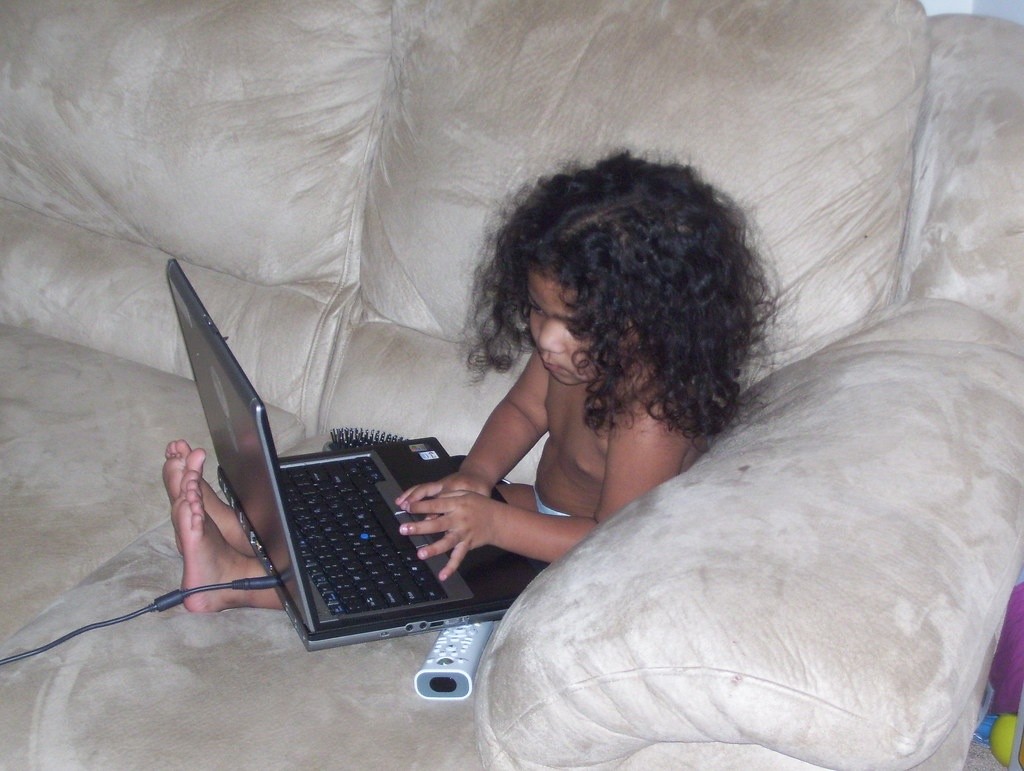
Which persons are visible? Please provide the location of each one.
[163,148,776,613]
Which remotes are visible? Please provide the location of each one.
[413,621,495,701]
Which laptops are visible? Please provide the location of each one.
[167,259,553,651]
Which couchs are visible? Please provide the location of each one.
[0,0,1024,771]
